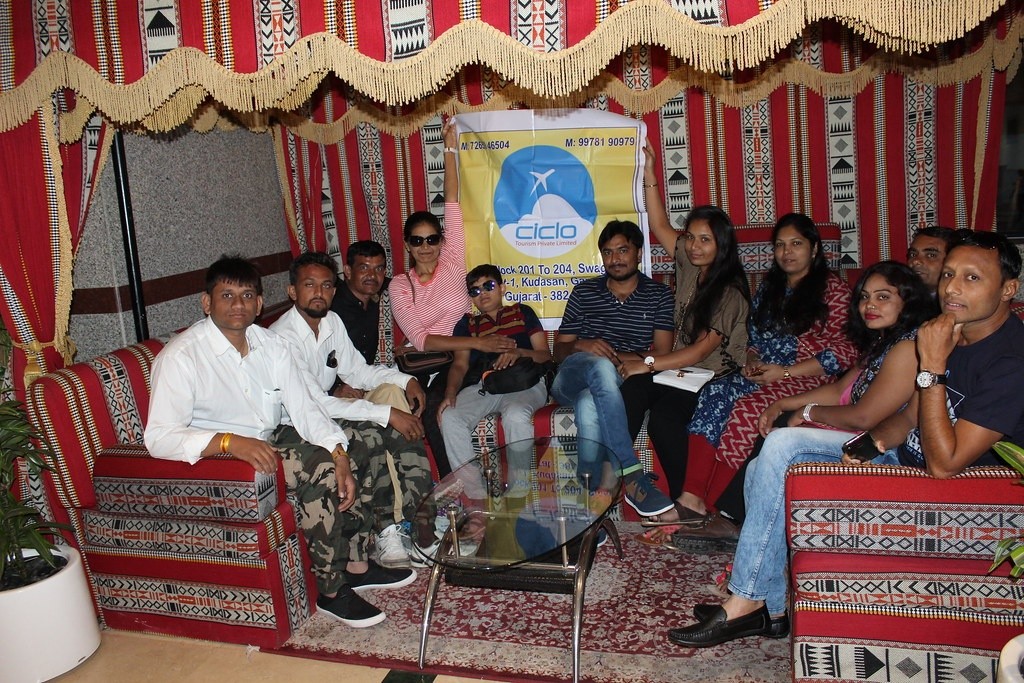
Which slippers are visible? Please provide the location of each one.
[663,535,679,551]
[634,525,685,545]
[458,515,486,540]
[641,500,709,526]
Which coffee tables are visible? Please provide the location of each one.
[409,437,625,683]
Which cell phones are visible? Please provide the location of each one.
[842,430,880,463]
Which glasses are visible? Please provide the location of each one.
[406,234,442,247]
[951,227,1009,257]
[468,280,500,297]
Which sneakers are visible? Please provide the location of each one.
[374,523,410,568]
[343,560,417,590]
[411,537,479,569]
[624,472,675,517]
[596,526,608,548]
[316,585,387,628]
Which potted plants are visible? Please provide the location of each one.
[1,321,102,683]
[987,439,1024,683]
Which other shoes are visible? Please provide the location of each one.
[672,509,744,555]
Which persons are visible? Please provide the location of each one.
[667,225,1024,648]
[616,135,753,543]
[143,257,418,627]
[642,212,861,549]
[265,251,474,567]
[550,219,675,548]
[329,241,484,541]
[387,113,516,530]
[435,264,552,538]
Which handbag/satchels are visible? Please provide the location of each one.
[479,356,547,396]
[393,346,456,373]
[653,365,715,394]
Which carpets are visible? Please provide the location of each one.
[244,514,792,682]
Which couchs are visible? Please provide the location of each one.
[30,223,1024,683]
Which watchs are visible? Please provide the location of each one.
[916,370,946,389]
[644,355,655,373]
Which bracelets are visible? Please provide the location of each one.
[219,433,233,452]
[803,403,818,421]
[784,366,791,378]
[332,450,348,460]
[443,148,457,153]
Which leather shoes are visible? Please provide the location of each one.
[668,606,772,647]
[694,604,790,640]
